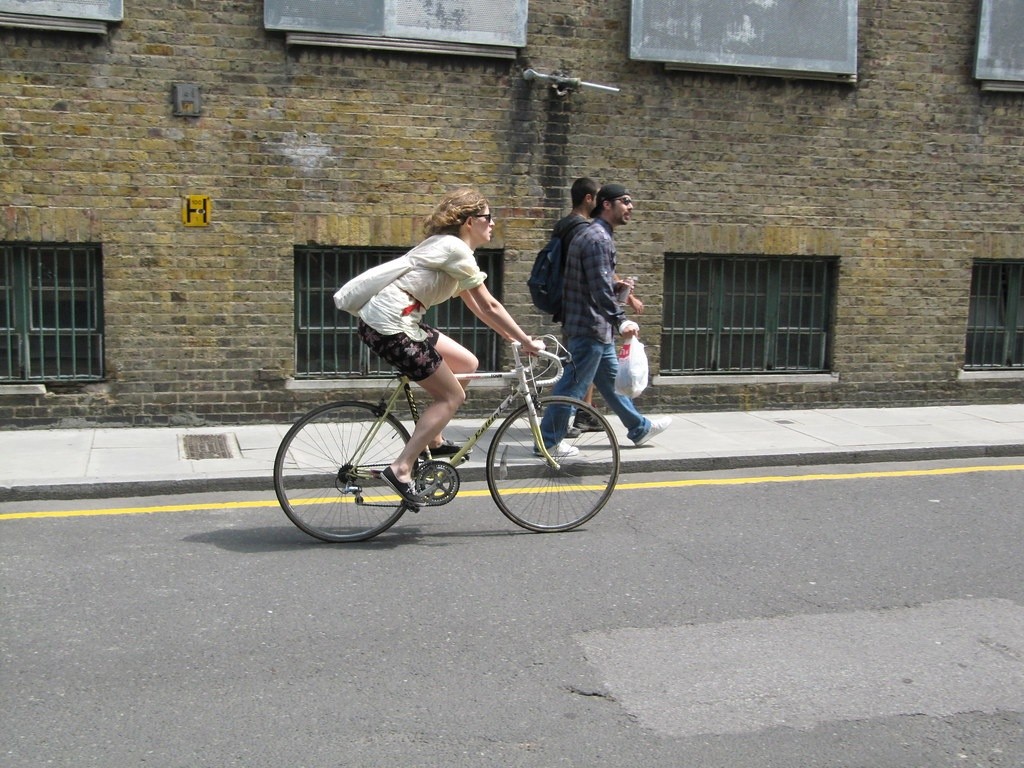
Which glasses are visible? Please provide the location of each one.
[474,213,491,224]
[614,196,632,206]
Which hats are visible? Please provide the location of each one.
[590,184,630,220]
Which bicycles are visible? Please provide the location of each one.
[269,332,620,548]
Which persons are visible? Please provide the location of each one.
[356,185,549,507]
[532,182,676,460]
[544,176,647,442]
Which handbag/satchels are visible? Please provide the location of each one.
[613,333,649,401]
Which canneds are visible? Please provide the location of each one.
[617,277,634,304]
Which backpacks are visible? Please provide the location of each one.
[526,220,592,324]
[333,255,412,318]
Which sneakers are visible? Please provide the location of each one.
[534,441,580,457]
[572,408,606,431]
[633,419,671,448]
[418,436,473,461]
[563,426,580,438]
[379,465,426,507]
